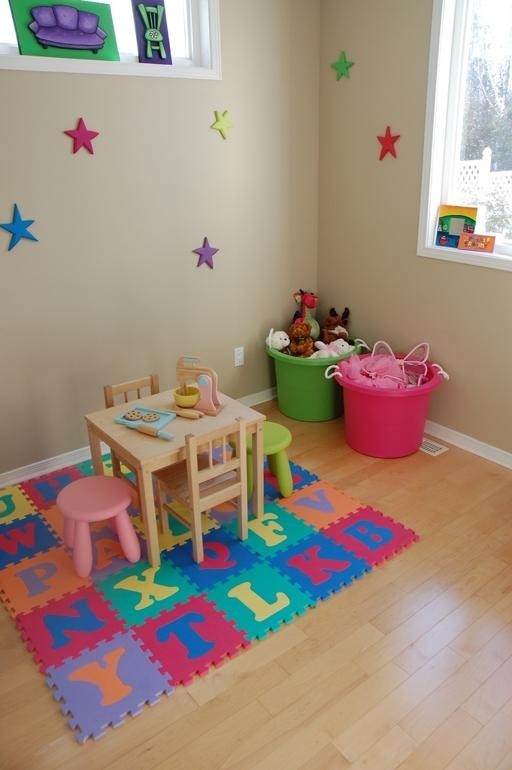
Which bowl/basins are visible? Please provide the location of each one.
[174,387,199,408]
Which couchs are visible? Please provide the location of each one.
[26,4,107,56]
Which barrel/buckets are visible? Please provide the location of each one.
[266,336,362,421]
[333,350,442,459]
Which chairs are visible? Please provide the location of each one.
[137,3,167,59]
[151,416,250,564]
[103,372,160,522]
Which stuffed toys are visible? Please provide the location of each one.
[266,288,356,360]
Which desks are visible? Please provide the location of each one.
[86,383,267,569]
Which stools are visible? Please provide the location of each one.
[56,475,142,577]
[230,420,293,498]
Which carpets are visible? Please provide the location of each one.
[0,452,424,746]
